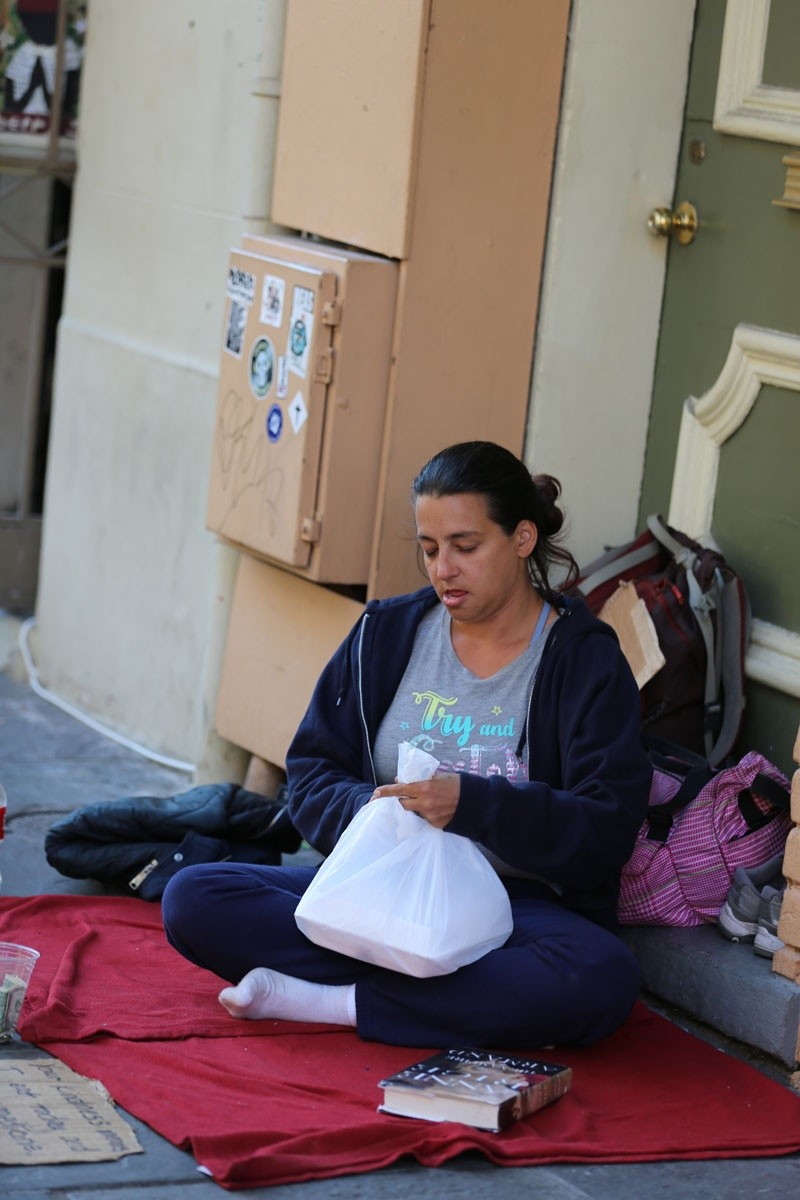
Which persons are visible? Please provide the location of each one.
[160,443,652,1054]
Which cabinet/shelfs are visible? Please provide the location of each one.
[205,232,398,584]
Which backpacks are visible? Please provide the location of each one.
[555,512,749,764]
[622,748,790,929]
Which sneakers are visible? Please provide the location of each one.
[752,884,787,961]
[718,852,785,944]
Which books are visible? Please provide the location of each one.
[376,1048,571,1136]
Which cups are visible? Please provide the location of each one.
[0,941,40,1042]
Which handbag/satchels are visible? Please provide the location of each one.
[293,741,513,979]
